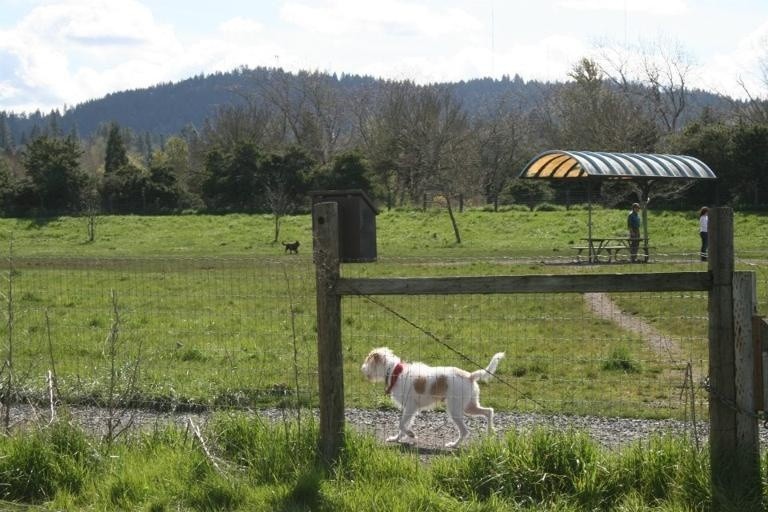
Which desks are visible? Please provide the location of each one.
[580,237,650,264]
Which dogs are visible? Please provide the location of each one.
[360,346,506,449]
[282,240,300,254]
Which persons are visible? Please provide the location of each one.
[699,206,709,261]
[627,203,641,262]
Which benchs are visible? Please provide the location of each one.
[570,247,656,262]
